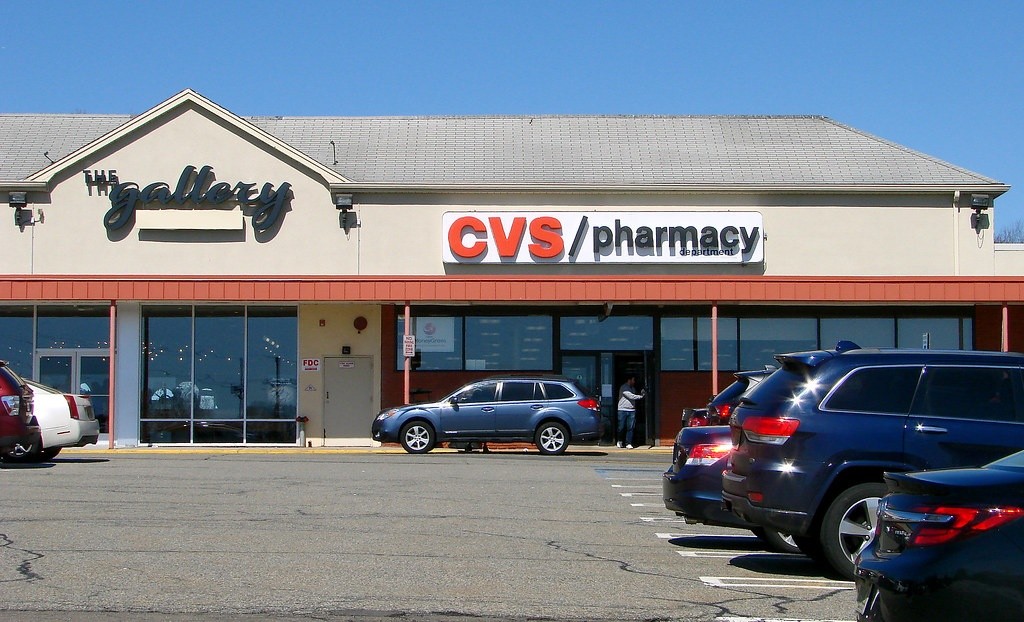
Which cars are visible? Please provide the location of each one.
[0,360,100,463]
[853,450,1024,622]
[662,363,807,553]
[146,422,255,443]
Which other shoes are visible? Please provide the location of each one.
[626,444,634,449]
[616,440,623,448]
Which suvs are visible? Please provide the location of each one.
[372,376,610,455]
[722,340,1024,581]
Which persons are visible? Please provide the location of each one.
[616,375,646,449]
[467,442,488,452]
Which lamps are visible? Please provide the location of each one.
[334,193,353,211]
[8,191,28,209]
[969,194,989,210]
